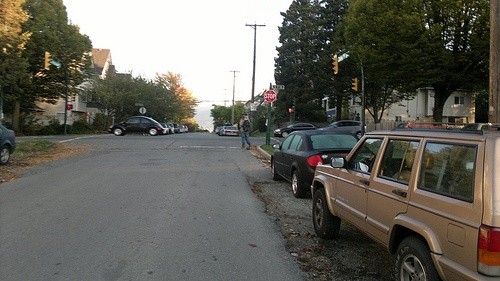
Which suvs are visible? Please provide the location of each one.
[310,124,500,281]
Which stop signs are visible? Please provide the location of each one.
[264,90,277,104]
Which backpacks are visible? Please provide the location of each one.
[241,119,250,132]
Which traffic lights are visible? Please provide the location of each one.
[67,104,74,110]
[352,77,358,93]
[288,108,292,112]
[332,54,339,74]
[71,92,76,102]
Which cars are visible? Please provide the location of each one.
[460,123,492,130]
[269,129,378,198]
[315,120,367,140]
[161,123,189,135]
[273,122,319,138]
[392,121,437,129]
[107,116,165,137]
[0,121,17,165]
[215,125,240,136]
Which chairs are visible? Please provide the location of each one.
[394,147,438,189]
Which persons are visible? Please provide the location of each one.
[239,111,251,149]
[356,113,360,121]
